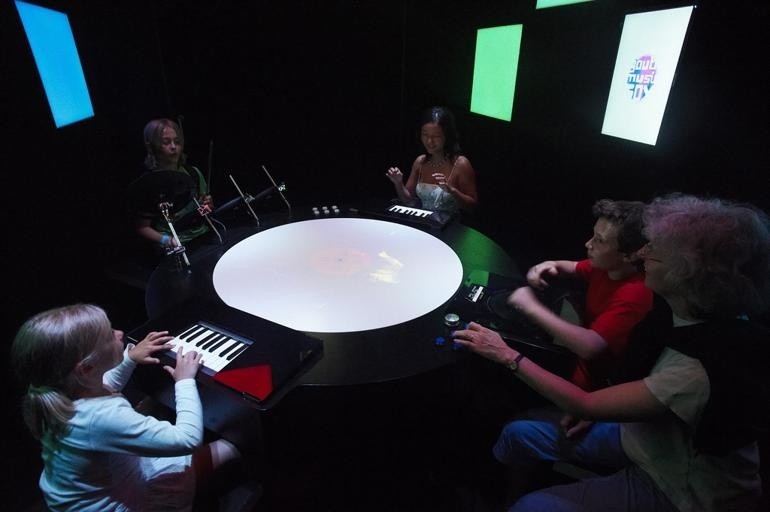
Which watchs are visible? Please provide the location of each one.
[509,353,526,372]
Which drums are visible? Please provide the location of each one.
[213,196,248,219]
[249,186,288,213]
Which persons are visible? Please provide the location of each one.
[13,305,257,512]
[509,200,660,393]
[138,120,212,270]
[452,194,769,511]
[386,108,477,224]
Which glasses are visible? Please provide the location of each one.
[636,242,670,266]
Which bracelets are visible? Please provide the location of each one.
[159,234,168,248]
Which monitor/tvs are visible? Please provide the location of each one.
[600,5,694,147]
[13,0,96,129]
[469,24,523,122]
[534,0,597,10]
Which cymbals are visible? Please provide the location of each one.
[125,169,197,219]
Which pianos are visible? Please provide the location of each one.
[164,318,254,382]
[388,201,454,232]
